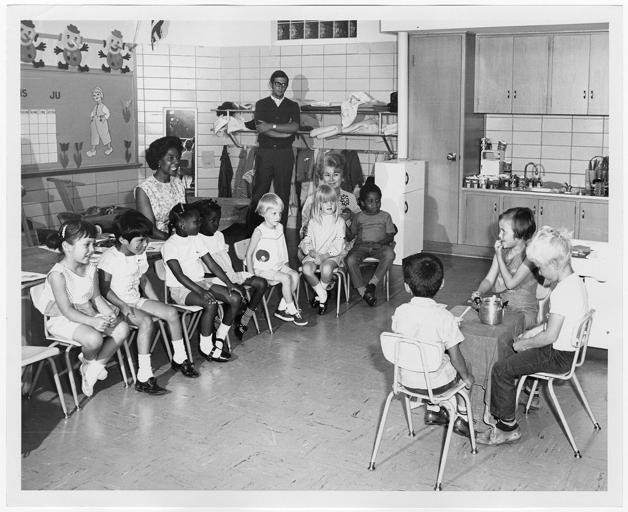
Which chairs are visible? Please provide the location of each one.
[536,284,550,324]
[368,332,478,491]
[515,309,601,458]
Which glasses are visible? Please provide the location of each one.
[272,81,289,88]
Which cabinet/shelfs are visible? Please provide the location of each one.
[211,109,397,154]
[551,31,609,115]
[503,193,576,238]
[465,192,499,247]
[579,199,608,242]
[409,31,484,244]
[474,34,549,115]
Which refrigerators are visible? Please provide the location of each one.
[372,157,426,266]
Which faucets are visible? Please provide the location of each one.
[524,162,538,179]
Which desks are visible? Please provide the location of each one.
[187,197,252,240]
[449,305,525,434]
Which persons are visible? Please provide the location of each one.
[391,252,478,439]
[475,224,589,446]
[247,193,308,325]
[247,69,300,235]
[133,137,187,238]
[471,207,540,331]
[43,199,267,397]
[298,150,396,315]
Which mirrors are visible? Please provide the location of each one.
[163,108,197,197]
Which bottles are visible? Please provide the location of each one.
[464,175,542,190]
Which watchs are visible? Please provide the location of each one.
[272,124,276,130]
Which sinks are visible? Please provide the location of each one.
[512,186,553,192]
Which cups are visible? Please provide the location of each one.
[585,168,608,197]
[479,299,504,326]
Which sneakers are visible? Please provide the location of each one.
[273,284,378,327]
[420,390,541,445]
[78,315,258,397]
